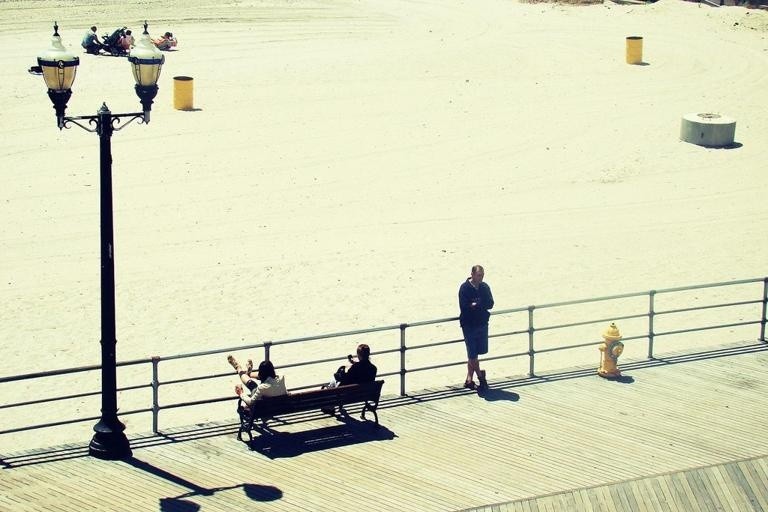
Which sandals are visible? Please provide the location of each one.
[464,380,488,391]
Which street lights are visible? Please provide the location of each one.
[37,20,165,459]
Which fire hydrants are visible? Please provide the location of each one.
[597,322,624,378]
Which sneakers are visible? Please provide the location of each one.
[227,355,240,371]
[246,359,253,374]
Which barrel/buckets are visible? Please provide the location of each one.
[625,35,643,66]
[173,76,194,110]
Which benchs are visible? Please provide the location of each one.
[236,379,386,444]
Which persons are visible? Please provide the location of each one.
[322,344,377,412]
[227,354,287,415]
[74,26,177,56]
[458,265,495,395]
